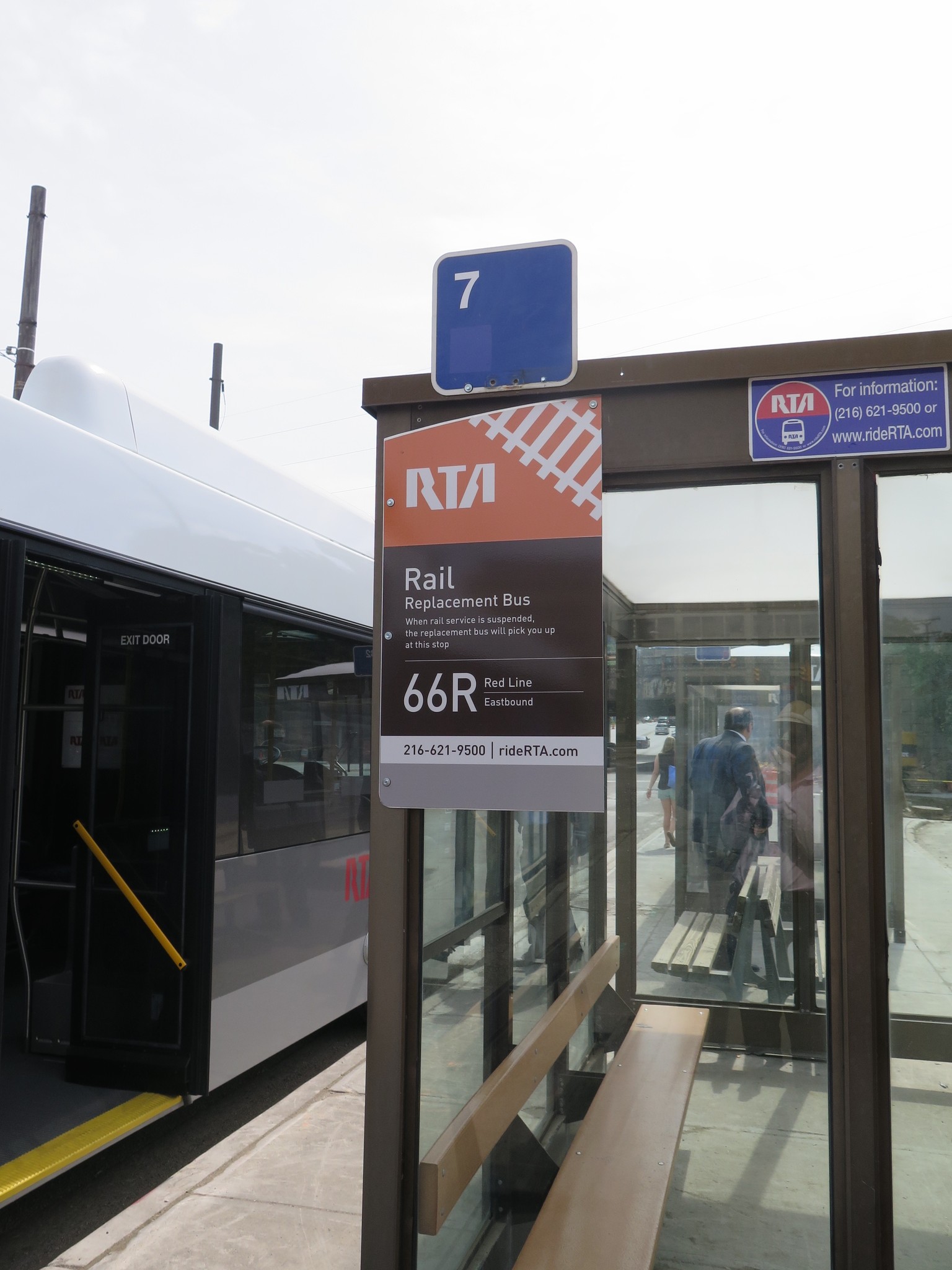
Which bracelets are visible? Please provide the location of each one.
[647,788,651,791]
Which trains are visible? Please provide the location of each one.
[1,352,601,1205]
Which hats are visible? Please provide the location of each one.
[772,700,812,725]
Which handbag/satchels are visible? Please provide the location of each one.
[667,751,676,790]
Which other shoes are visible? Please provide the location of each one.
[664,843,670,848]
[667,831,676,848]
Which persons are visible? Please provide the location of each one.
[687,707,771,908]
[646,736,676,848]
[763,700,825,930]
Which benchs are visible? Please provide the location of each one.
[412,935,714,1270]
[649,903,740,983]
[517,843,599,958]
[759,862,827,1007]
